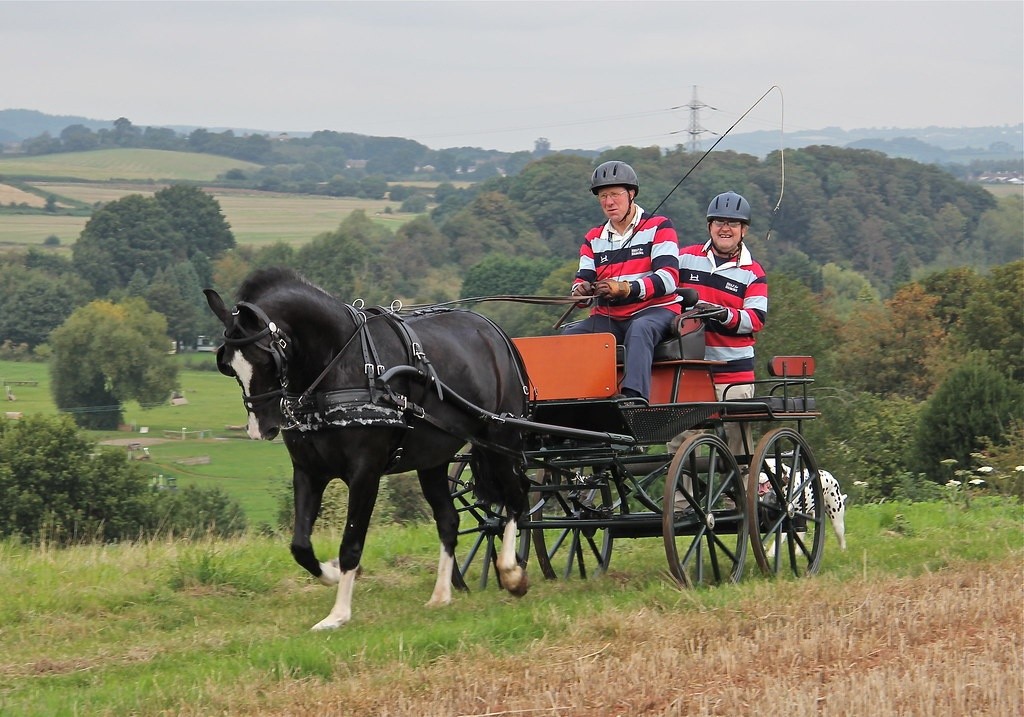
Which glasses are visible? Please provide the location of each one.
[599,189,627,197]
[712,220,742,228]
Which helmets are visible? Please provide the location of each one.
[706,190,752,225]
[589,160,640,198]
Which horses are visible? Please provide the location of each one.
[205,268,531,635]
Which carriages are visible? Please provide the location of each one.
[201,263,827,635]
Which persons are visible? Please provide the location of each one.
[666,190,768,510]
[560,160,680,404]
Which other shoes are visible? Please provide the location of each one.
[612,394,627,406]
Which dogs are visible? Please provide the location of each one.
[758,458,848,556]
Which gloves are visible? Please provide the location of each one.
[596,279,631,299]
[698,303,728,322]
[572,280,595,304]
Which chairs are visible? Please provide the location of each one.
[567,286,727,360]
[718,355,817,412]
